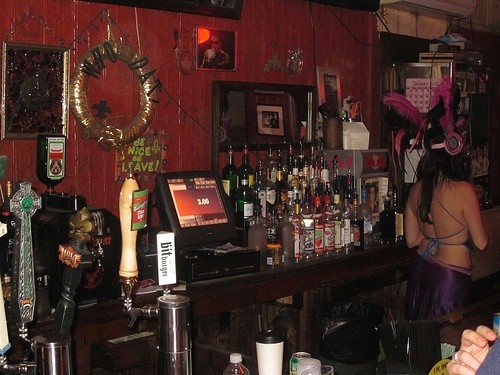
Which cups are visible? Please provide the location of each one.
[297,357,335,375]
[255,328,285,375]
[30,332,74,375]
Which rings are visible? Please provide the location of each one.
[452,351,460,362]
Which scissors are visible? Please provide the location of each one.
[350,101,362,118]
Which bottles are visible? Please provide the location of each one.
[220,141,406,266]
[34,133,66,193]
[222,353,251,375]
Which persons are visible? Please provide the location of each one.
[266,113,275,126]
[404,128,488,321]
[201,31,229,68]
[366,186,377,212]
[447,324,500,375]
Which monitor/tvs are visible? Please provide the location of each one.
[155,170,239,249]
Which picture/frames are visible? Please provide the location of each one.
[317,65,343,117]
[194,25,239,70]
[256,103,286,136]
[2,42,72,141]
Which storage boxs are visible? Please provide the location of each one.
[324,149,390,176]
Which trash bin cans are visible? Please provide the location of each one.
[318,299,384,375]
